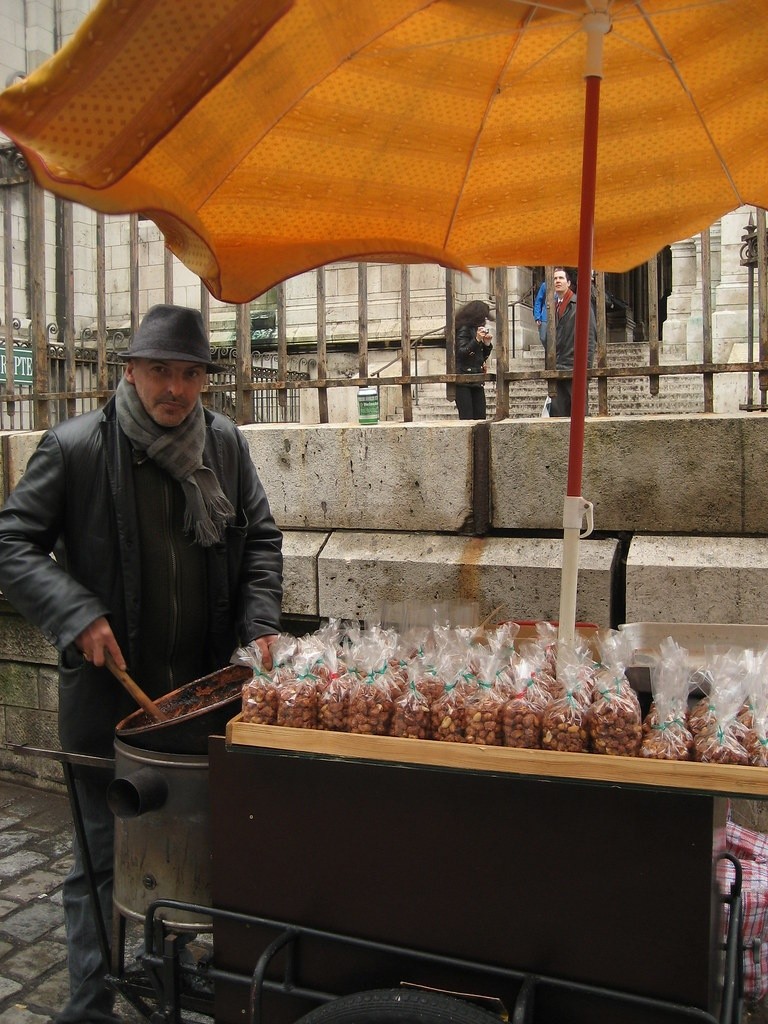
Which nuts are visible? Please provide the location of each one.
[241,656,768,767]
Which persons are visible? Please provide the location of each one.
[543,271,597,416]
[455,300,494,419]
[592,282,614,311]
[534,265,565,348]
[0,303,285,1024]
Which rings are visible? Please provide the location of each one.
[83,653,92,659]
[267,641,272,646]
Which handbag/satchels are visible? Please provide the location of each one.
[725,820,768,1004]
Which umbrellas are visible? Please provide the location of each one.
[0,0,768,665]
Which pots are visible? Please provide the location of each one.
[115,664,253,738]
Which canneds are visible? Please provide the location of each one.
[357,387,378,424]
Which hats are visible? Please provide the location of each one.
[117,304,227,374]
